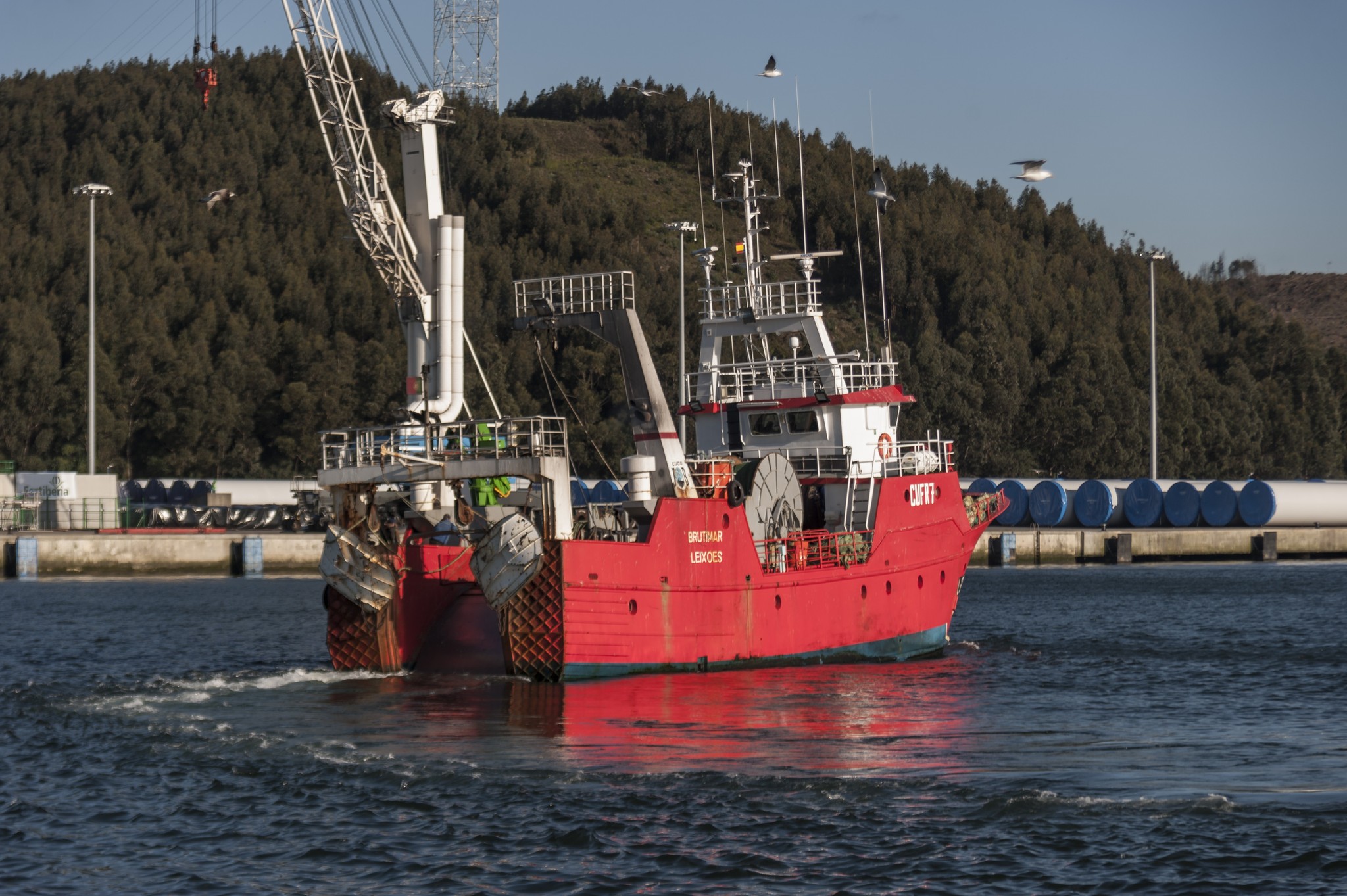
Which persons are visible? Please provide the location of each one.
[428,514,467,546]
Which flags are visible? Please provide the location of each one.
[736,243,744,254]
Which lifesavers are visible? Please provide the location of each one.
[878,434,892,459]
[727,480,744,507]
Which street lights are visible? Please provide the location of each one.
[70,180,114,476]
[662,220,699,452]
[1137,252,1168,479]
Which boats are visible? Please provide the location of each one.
[316,82,1012,684]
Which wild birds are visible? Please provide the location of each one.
[1009,159,1053,182]
[867,166,896,216]
[198,188,236,210]
[756,54,784,78]
[614,85,668,96]
